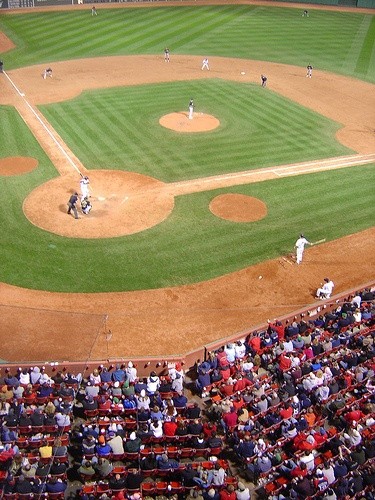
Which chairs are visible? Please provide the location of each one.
[0,299,375,500]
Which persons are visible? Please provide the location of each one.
[293,234,313,264]
[315,278,334,299]
[44,67,53,79]
[188,98,194,119]
[164,47,170,62]
[67,174,92,219]
[306,65,312,78]
[0,59,3,73]
[91,6,97,16]
[260,74,267,87]
[304,10,308,17]
[202,59,210,71]
[0,286,375,500]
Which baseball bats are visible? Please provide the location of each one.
[313,238,325,245]
